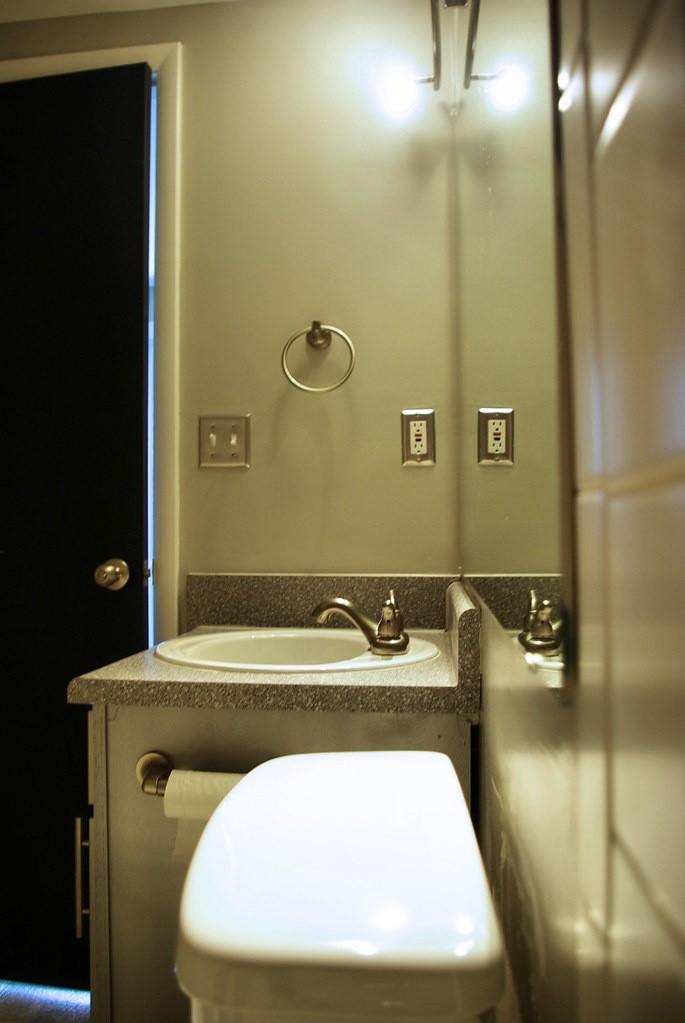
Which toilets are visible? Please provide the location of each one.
[175,752,509,1023]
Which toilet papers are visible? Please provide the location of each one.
[163,767,248,860]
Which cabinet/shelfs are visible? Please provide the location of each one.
[88,703,471,1023]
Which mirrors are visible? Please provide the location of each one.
[449,0,581,709]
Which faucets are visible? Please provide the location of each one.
[314,590,411,654]
[516,588,566,650]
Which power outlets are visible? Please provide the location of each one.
[400,410,436,467]
[477,408,514,465]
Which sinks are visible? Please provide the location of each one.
[513,634,566,672]
[156,628,442,672]
